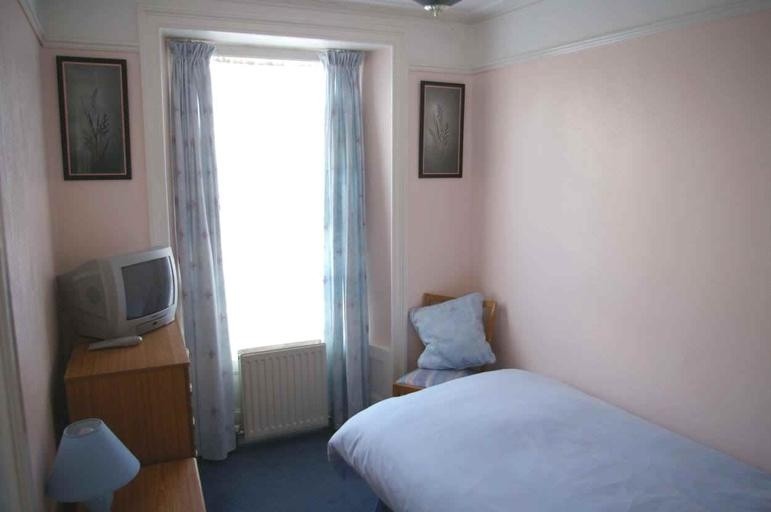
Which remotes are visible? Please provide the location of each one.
[88,335,142,351]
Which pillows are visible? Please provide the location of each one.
[408,292,496,370]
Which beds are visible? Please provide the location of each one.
[327,368,771,512]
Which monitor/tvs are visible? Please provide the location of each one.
[56,246,178,340]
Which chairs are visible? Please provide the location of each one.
[392,292,497,397]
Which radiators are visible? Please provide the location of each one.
[238,339,329,443]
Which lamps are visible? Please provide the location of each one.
[46,418,141,512]
[414,0,462,16]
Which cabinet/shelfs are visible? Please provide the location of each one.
[62,314,207,512]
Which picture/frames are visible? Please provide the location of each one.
[417,81,465,179]
[56,55,132,180]
